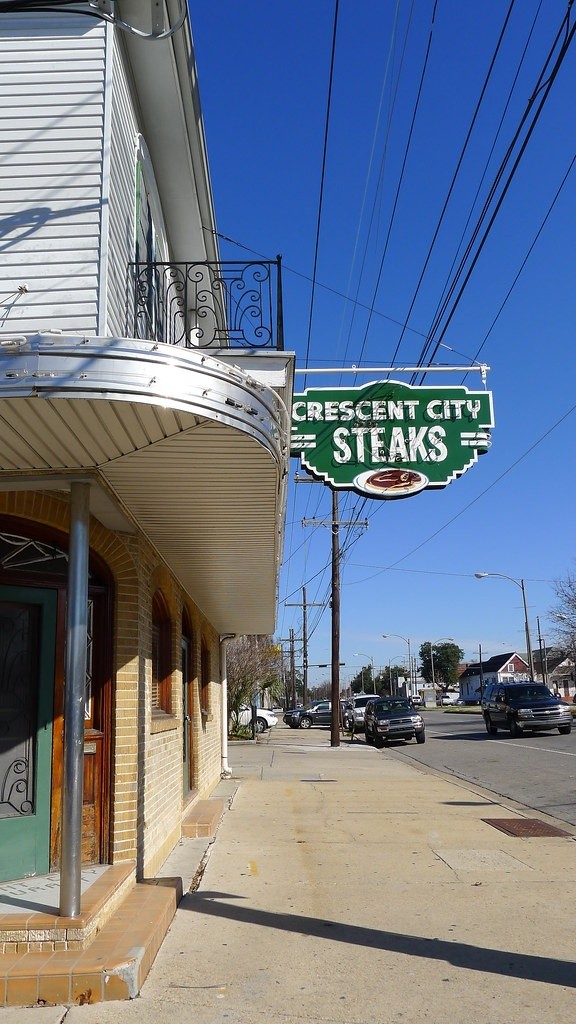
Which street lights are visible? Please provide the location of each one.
[383,634,413,696]
[352,653,377,694]
[536,638,548,682]
[389,655,405,696]
[431,638,454,688]
[474,573,535,683]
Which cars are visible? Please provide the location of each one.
[232,702,279,732]
[296,702,303,708]
[273,705,283,713]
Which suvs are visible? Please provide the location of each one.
[347,694,388,734]
[283,699,353,730]
[481,681,573,738]
[412,695,422,705]
[364,696,426,749]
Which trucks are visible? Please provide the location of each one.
[436,692,459,706]
